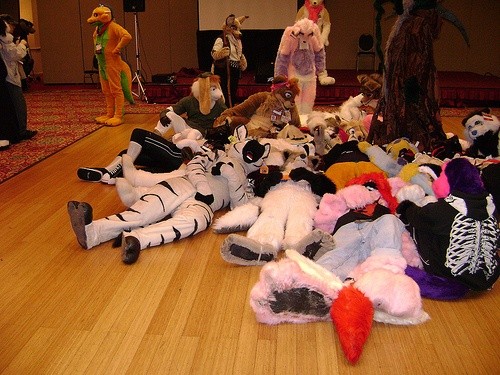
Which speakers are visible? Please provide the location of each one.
[356,33,375,70]
[122,0,146,12]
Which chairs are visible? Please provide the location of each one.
[83,54,100,89]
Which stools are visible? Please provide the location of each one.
[356,51,377,73]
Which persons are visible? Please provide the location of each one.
[0,14,39,150]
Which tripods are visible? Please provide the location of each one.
[131,13,149,104]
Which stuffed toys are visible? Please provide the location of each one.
[211,14,249,108]
[272,20,336,115]
[295,0,331,47]
[366,0,471,154]
[87,3,136,127]
[66,70,500,361]
